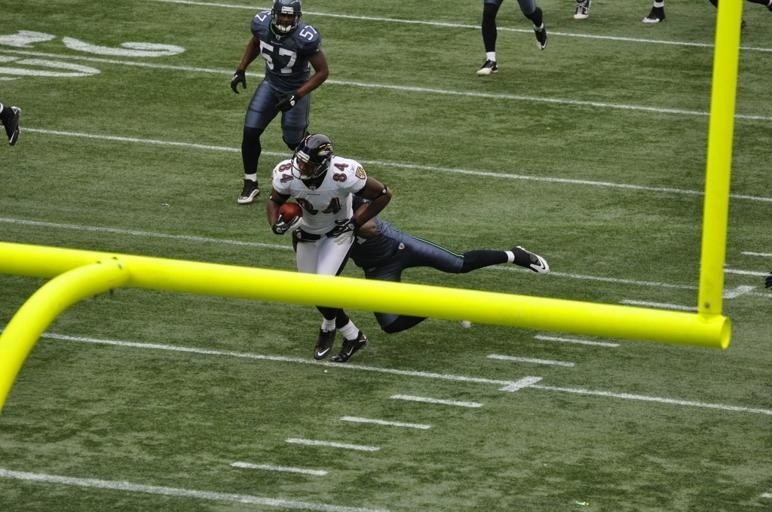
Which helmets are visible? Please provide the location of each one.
[291,134,333,180]
[270,0,302,36]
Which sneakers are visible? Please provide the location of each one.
[6,105,21,147]
[477,59,498,76]
[511,245,550,276]
[534,21,547,50]
[331,330,368,362]
[642,6,665,24]
[237,178,260,204]
[574,0,591,19]
[313,326,337,359]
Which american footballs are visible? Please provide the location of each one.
[278,203,302,224]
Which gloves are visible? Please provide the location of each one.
[231,70,246,94]
[324,218,354,238]
[272,214,302,235]
[275,91,298,111]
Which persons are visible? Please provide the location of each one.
[477,1,548,78]
[262,131,395,366]
[342,183,551,338]
[0,99,24,147]
[229,0,331,205]
[572,0,667,26]
[709,0,771,30]
